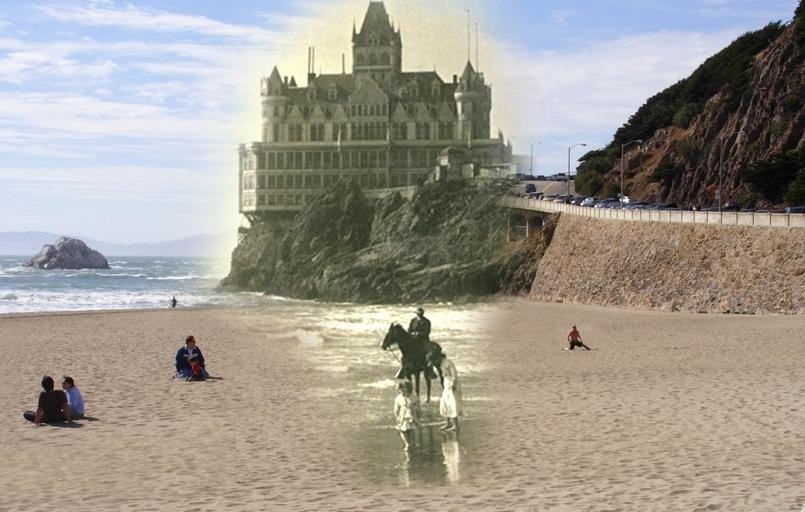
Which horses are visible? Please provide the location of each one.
[379,323,445,403]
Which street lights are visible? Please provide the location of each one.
[567,143,587,196]
[530,141,542,180]
[621,139,645,208]
[717,130,746,212]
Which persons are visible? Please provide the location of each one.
[561,324,591,353]
[172,334,209,381]
[187,355,206,381]
[394,381,424,454]
[426,349,464,432]
[172,296,177,310]
[408,307,437,380]
[60,376,85,420]
[23,374,75,427]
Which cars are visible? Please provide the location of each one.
[524,173,744,212]
